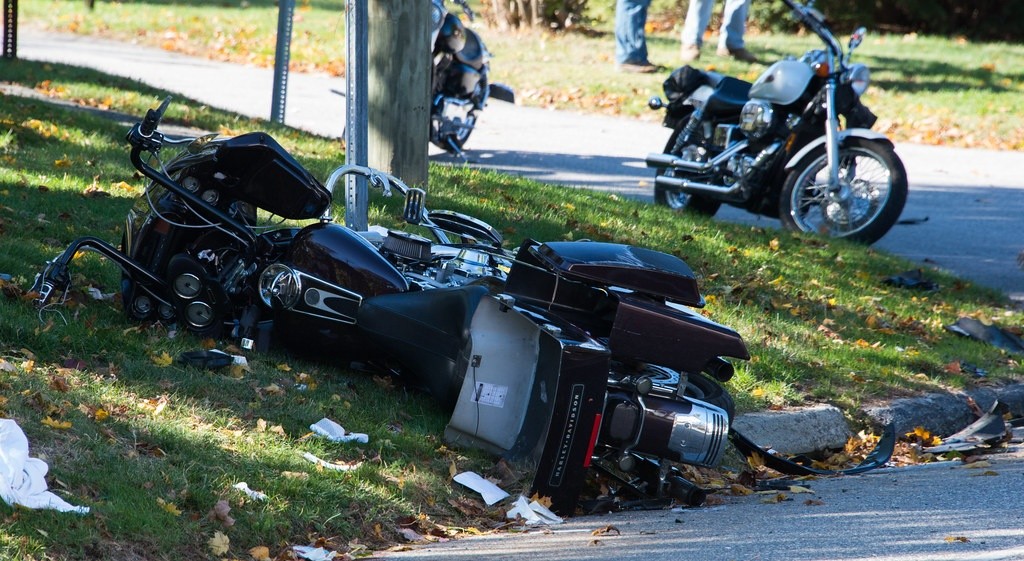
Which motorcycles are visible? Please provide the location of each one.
[25,91,751,512]
[645,0,908,247]
[331,0,514,166]
